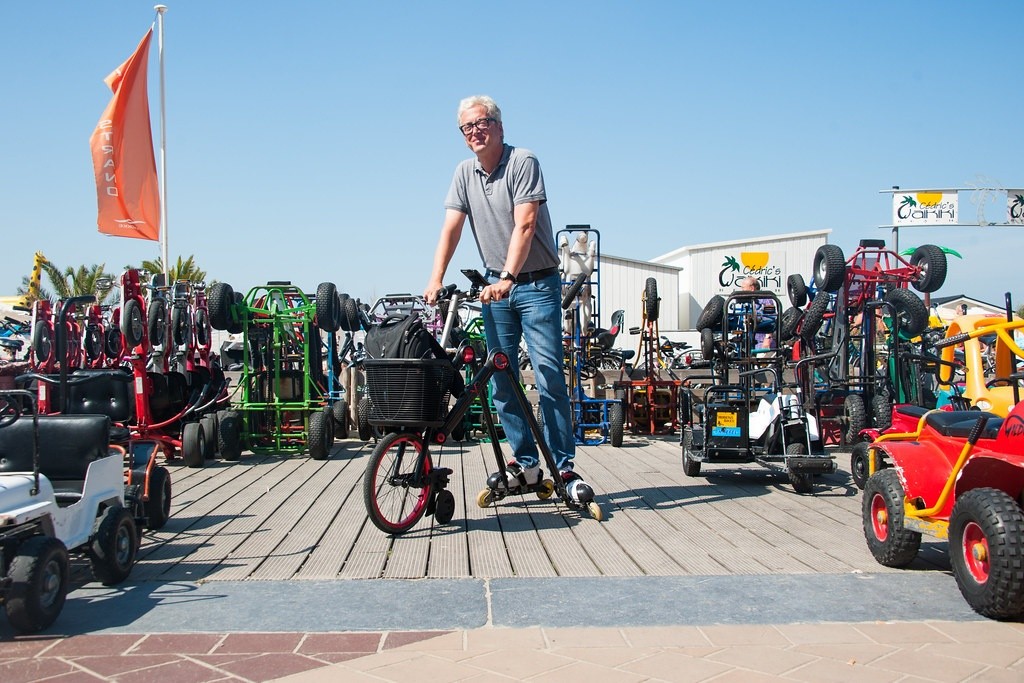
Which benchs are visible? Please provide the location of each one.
[0,412,110,501]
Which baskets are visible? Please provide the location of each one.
[363,357,447,428]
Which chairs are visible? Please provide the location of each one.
[67,362,229,444]
[748,395,820,441]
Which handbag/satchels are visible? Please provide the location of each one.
[363,314,465,422]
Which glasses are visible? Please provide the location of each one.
[459,118,502,135]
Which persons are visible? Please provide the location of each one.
[740,276,779,351]
[423,96,594,504]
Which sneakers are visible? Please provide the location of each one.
[557,465,595,502]
[486,457,541,490]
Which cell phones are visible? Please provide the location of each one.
[461,269,490,286]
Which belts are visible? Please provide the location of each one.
[485,266,559,284]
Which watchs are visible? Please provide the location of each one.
[499,270,517,284]
[765,335,772,340]
